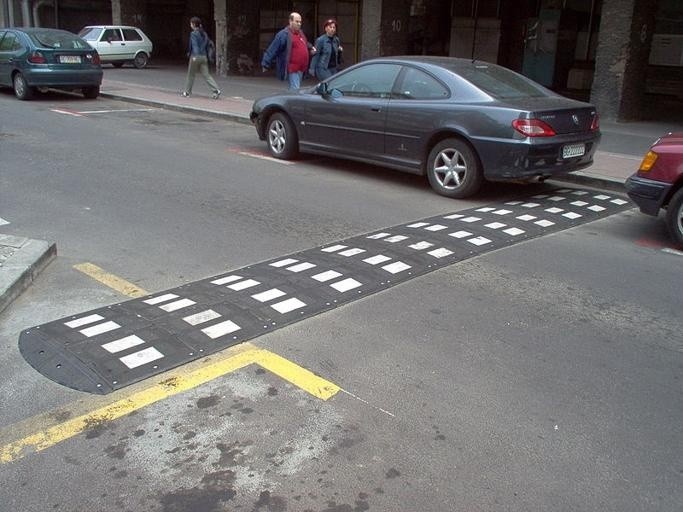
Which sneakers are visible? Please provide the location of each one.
[211,90,220,100]
[180,91,190,97]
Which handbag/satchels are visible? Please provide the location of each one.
[206,39,215,64]
[337,51,345,64]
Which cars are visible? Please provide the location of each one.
[622,130,682,250]
[0,24,102,102]
[247,55,602,200]
[77,26,154,69]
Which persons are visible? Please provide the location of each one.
[179,16,221,99]
[260,12,317,92]
[308,19,342,84]
